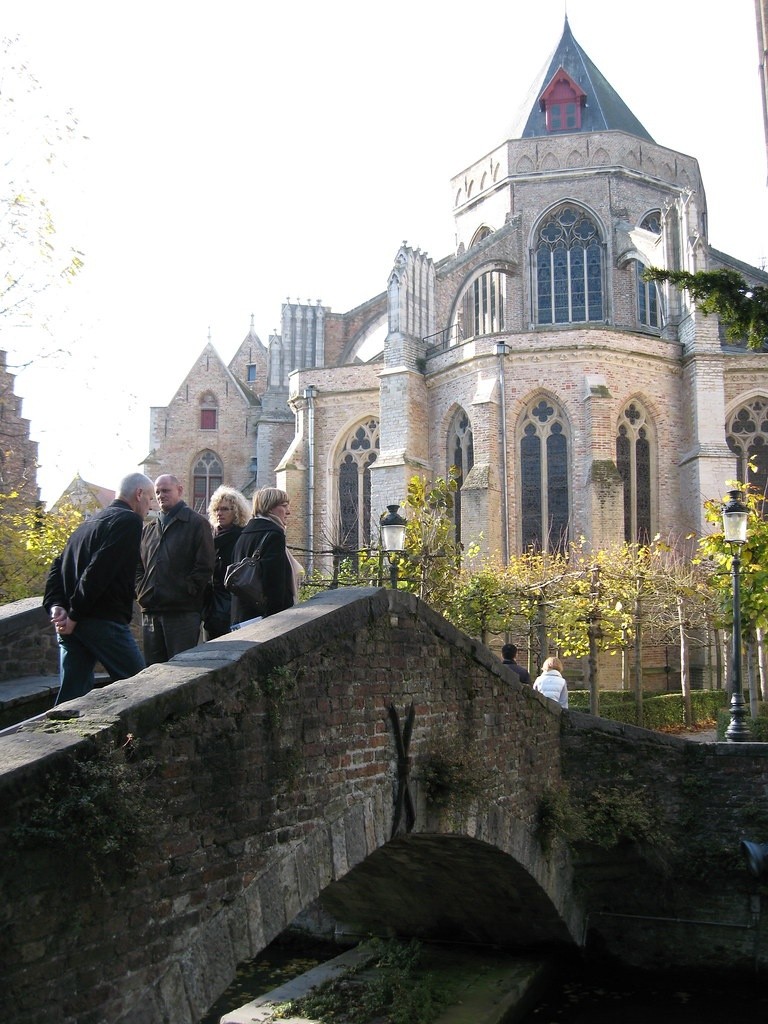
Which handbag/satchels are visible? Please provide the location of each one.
[223,531,267,612]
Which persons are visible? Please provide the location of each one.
[201,485,252,641]
[229,488,294,632]
[133,474,215,667]
[42,472,156,707]
[532,656,568,710]
[502,644,531,684]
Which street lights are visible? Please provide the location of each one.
[721,489,758,742]
[380,505,409,588]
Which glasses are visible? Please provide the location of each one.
[213,506,234,512]
[276,504,291,509]
